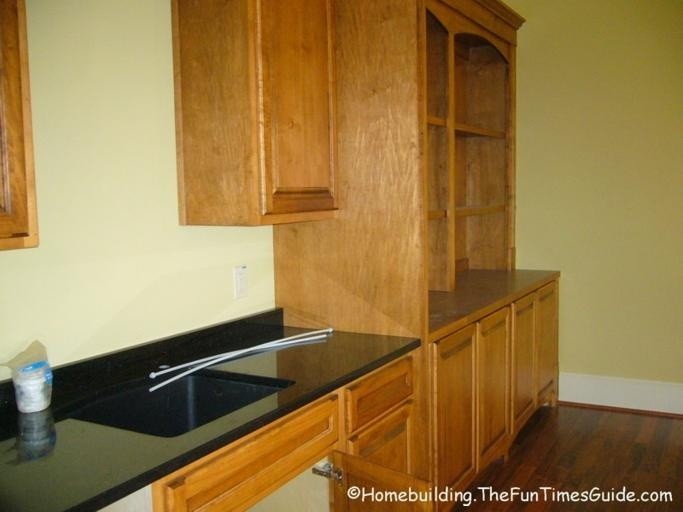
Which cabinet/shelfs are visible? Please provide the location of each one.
[0,0,562,512]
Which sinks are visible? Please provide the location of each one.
[58,367,297,438]
